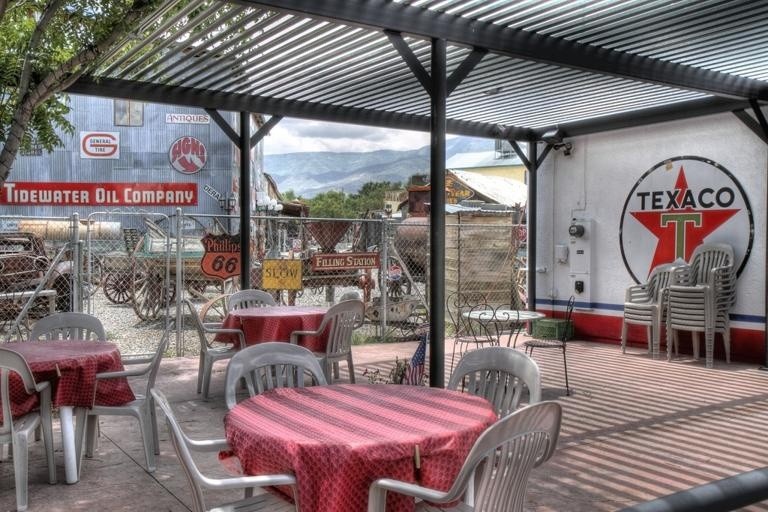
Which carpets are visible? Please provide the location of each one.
[334,342,768,512]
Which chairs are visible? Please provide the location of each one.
[228,289,277,310]
[225,343,327,409]
[665,244,737,368]
[447,347,540,499]
[185,299,258,402]
[468,304,519,392]
[31,312,104,454]
[76,322,174,480]
[621,263,686,356]
[0,348,57,512]
[524,295,575,395]
[289,300,365,384]
[334,292,360,378]
[151,389,299,512]
[446,291,496,393]
[368,401,563,512]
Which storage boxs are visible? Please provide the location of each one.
[535,318,573,341]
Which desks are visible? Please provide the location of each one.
[0,341,137,484]
[463,310,545,393]
[214,307,330,386]
[219,384,496,512]
